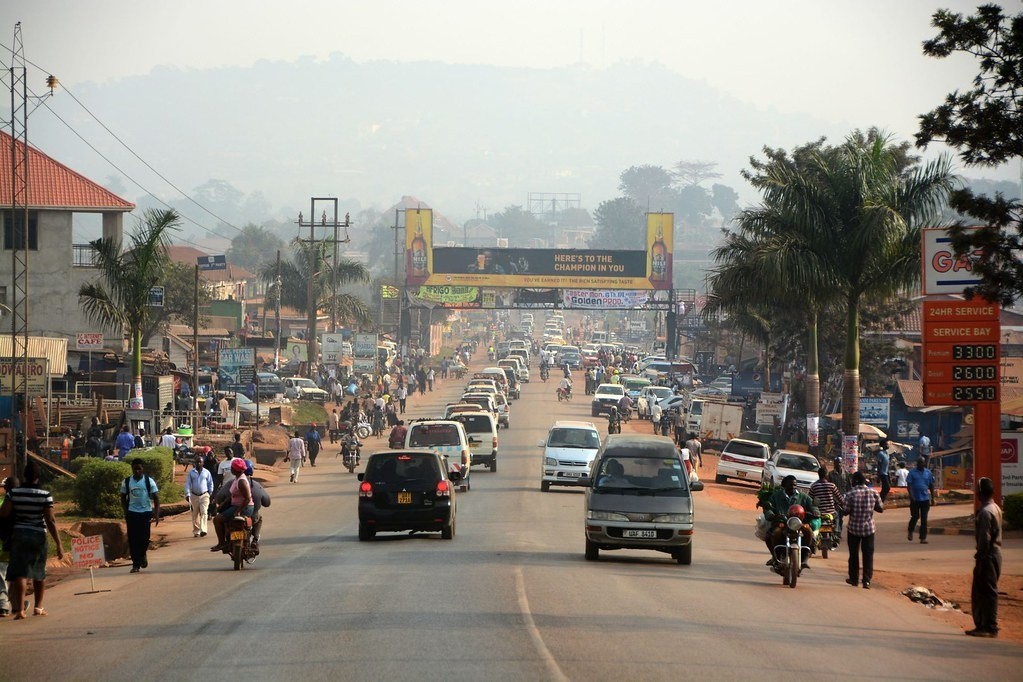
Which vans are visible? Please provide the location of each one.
[583,433,704,565]
[449,411,499,472]
[393,418,473,492]
[538,421,602,492]
[443,390,500,430]
[357,449,462,541]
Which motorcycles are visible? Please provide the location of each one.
[328,412,370,474]
[220,515,260,571]
[756,500,836,589]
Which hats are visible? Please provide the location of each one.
[232,458,247,473]
[311,422,317,426]
[896,461,905,467]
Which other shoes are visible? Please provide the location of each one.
[293,480,298,482]
[908,531,913,540]
[24,600,30,611]
[846,578,859,586]
[801,560,809,568]
[141,555,147,568]
[2,610,11,617]
[965,628,994,638]
[251,542,258,548]
[200,532,206,537]
[863,582,870,588]
[290,477,293,482]
[130,568,139,573]
[921,539,928,544]
[766,559,773,566]
[210,544,223,551]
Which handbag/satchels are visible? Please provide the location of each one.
[688,469,698,482]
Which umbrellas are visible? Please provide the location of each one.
[858,424,887,440]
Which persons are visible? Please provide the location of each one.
[134,429,146,447]
[86,416,117,457]
[204,446,271,552]
[244,313,250,329]
[158,426,177,451]
[285,383,299,399]
[286,429,305,483]
[328,343,471,465]
[120,459,160,573]
[247,379,257,401]
[186,460,214,537]
[231,433,245,458]
[71,431,85,459]
[288,345,305,361]
[0,461,65,620]
[467,250,506,274]
[460,315,1001,637]
[315,371,325,388]
[59,432,68,468]
[66,428,75,458]
[162,389,229,425]
[304,423,323,466]
[117,426,135,461]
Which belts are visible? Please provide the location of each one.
[191,491,207,496]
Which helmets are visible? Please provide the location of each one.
[244,459,254,476]
[788,505,804,521]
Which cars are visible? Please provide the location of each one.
[256,373,287,399]
[715,438,772,484]
[342,341,397,367]
[541,310,583,401]
[207,391,269,425]
[581,342,623,368]
[492,392,512,428]
[762,449,821,495]
[591,356,733,435]
[453,313,540,400]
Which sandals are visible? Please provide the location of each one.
[32,608,49,617]
[14,615,26,620]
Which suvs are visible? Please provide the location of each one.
[284,378,329,407]
